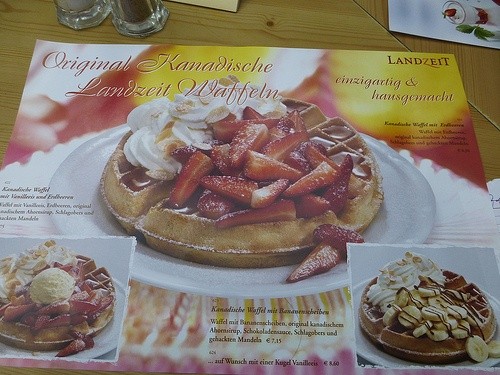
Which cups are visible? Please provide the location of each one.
[441,0,488,26]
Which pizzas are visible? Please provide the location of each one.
[100,74,384,267]
[0,239,116,350]
[358,251,496,364]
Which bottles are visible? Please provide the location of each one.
[53,0,111,30]
[108,0,170,37]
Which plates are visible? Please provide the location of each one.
[352,278,500,368]
[0,278,126,359]
[45,125,436,298]
